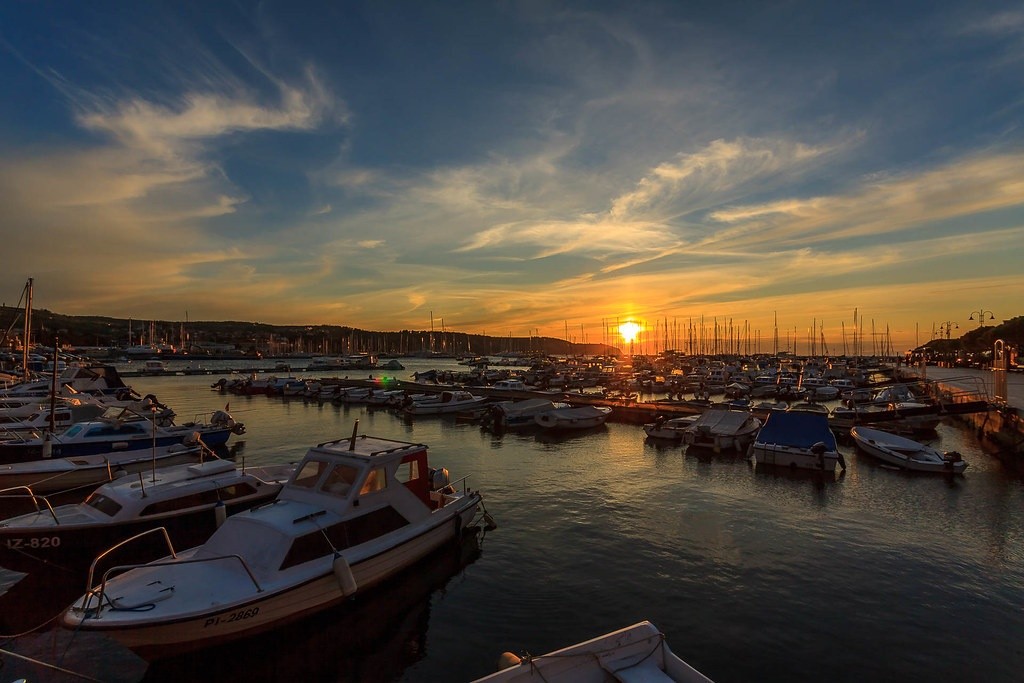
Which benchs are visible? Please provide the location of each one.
[602,653,674,683]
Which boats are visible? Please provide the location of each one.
[850,425,970,476]
[461,620,717,683]
[57,417,496,664]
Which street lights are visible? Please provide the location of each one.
[940,320,960,340]
[935,329,948,340]
[968,309,997,328]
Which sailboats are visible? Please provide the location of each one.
[0,273,945,575]
